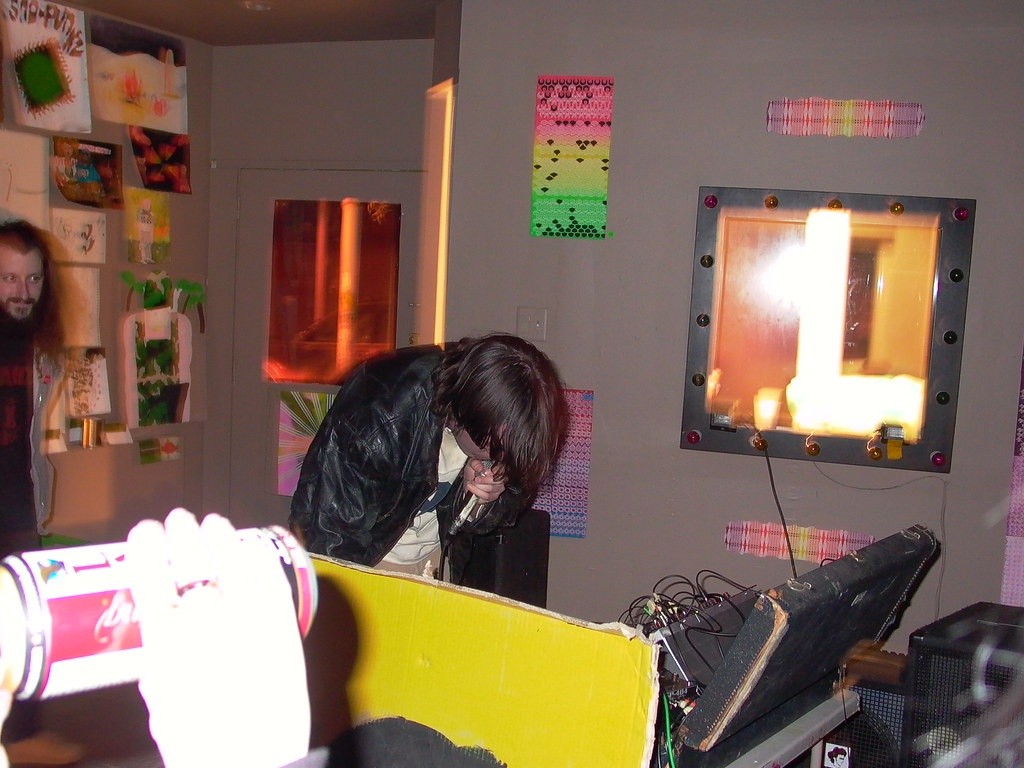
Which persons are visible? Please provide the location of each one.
[289,332,569,583]
[0,220,64,562]
[0,507,328,768]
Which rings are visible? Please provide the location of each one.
[175,580,220,596]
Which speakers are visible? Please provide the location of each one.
[901,600,1024,768]
[815,647,904,768]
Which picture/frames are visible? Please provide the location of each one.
[681,184,978,477]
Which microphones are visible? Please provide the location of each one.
[446,461,494,539]
